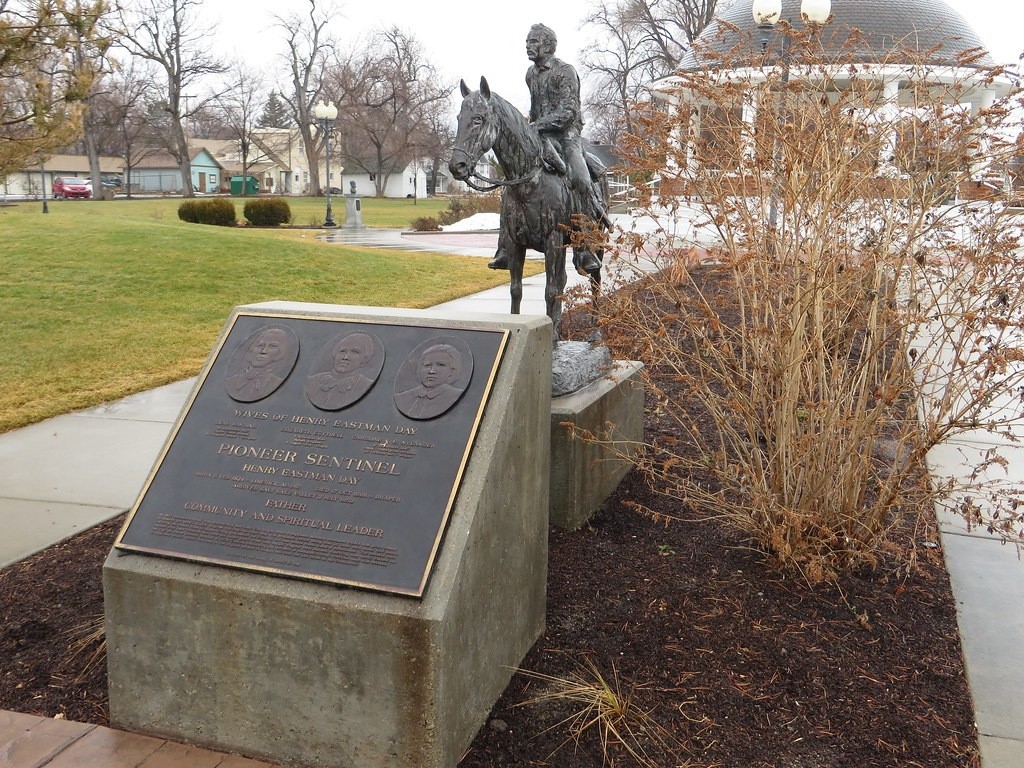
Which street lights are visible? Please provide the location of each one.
[311,100,342,226]
[752,0,832,260]
[27,114,54,213]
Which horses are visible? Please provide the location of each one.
[448,76,612,343]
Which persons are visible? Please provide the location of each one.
[350,181,356,193]
[487,24,603,270]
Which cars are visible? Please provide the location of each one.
[52,177,92,199]
[85,176,122,197]
